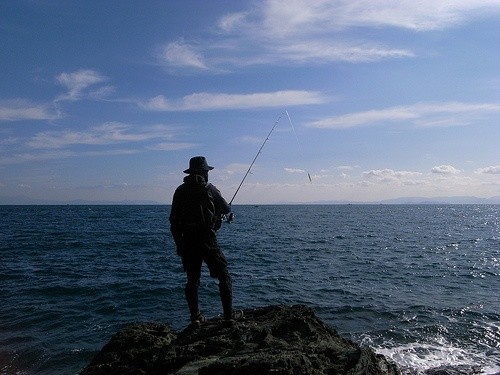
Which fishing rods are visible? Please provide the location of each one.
[220,102,287,225]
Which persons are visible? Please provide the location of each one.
[170,156,243,325]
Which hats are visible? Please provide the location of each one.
[182,156,214,173]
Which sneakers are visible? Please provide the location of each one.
[222,309,244,328]
[190,314,207,328]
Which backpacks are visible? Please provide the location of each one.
[174,175,216,232]
